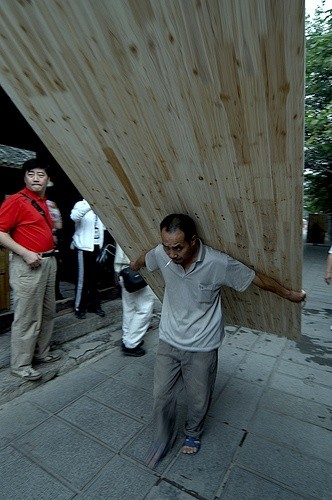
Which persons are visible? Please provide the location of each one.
[324,245,332,285]
[0,158,62,381]
[128,213,307,468]
[41,177,154,357]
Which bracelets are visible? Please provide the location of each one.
[326,272,332,273]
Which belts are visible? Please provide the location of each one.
[12,251,55,257]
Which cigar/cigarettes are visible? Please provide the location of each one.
[165,259,172,268]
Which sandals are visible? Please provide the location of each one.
[182,434,201,455]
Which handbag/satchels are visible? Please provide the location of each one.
[118,267,148,293]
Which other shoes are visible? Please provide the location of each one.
[124,346,146,357]
[122,340,144,347]
[33,353,61,362]
[75,307,85,319]
[10,368,41,380]
[55,296,66,301]
[95,307,105,316]
